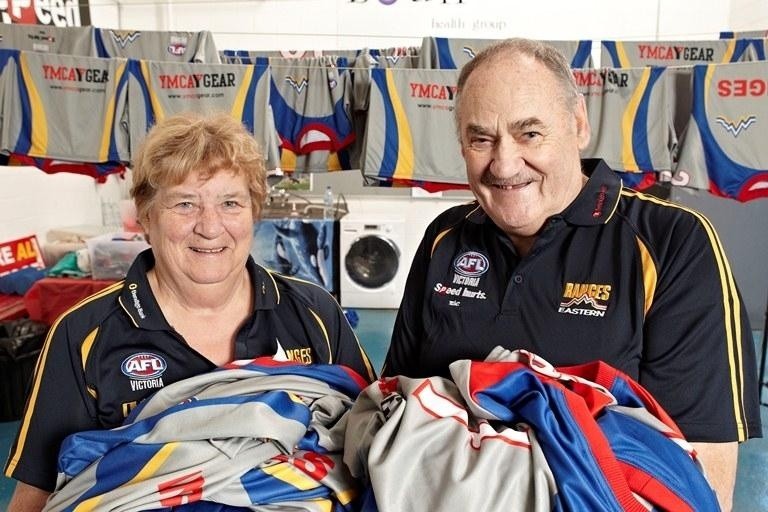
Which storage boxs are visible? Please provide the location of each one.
[90,234,148,280]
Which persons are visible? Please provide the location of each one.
[3,111,378,511]
[379,38,763,512]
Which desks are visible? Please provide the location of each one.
[25,276,119,324]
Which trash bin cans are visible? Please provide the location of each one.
[0,320,50,424]
